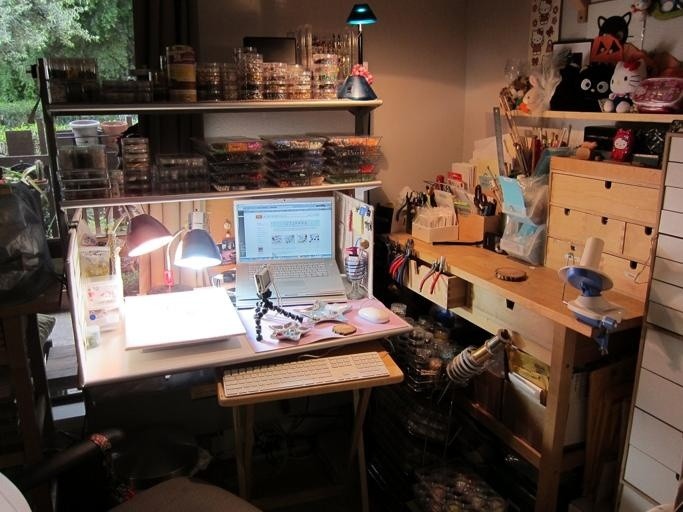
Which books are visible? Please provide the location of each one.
[122,286,247,352]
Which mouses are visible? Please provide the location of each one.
[360,305,390,323]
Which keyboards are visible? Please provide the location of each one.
[222,348,390,397]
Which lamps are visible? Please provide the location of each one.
[111,202,222,297]
[557,236,626,331]
[338,3,379,99]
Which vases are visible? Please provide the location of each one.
[55,119,128,149]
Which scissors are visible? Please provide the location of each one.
[474,184,487,215]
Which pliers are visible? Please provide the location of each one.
[396,192,413,221]
[420,255,446,294]
[383,235,417,286]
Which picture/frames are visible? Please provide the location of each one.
[551,38,594,65]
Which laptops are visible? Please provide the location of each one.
[233,196,345,301]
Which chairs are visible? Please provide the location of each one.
[0,424,266,511]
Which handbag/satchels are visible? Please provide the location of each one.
[632,76,683,114]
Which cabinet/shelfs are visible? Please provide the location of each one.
[615,131,682,512]
[38,96,384,223]
[543,154,663,316]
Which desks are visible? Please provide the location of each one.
[385,227,650,511]
[65,197,415,510]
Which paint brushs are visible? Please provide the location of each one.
[497,96,572,176]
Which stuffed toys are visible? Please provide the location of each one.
[522,36,648,114]
